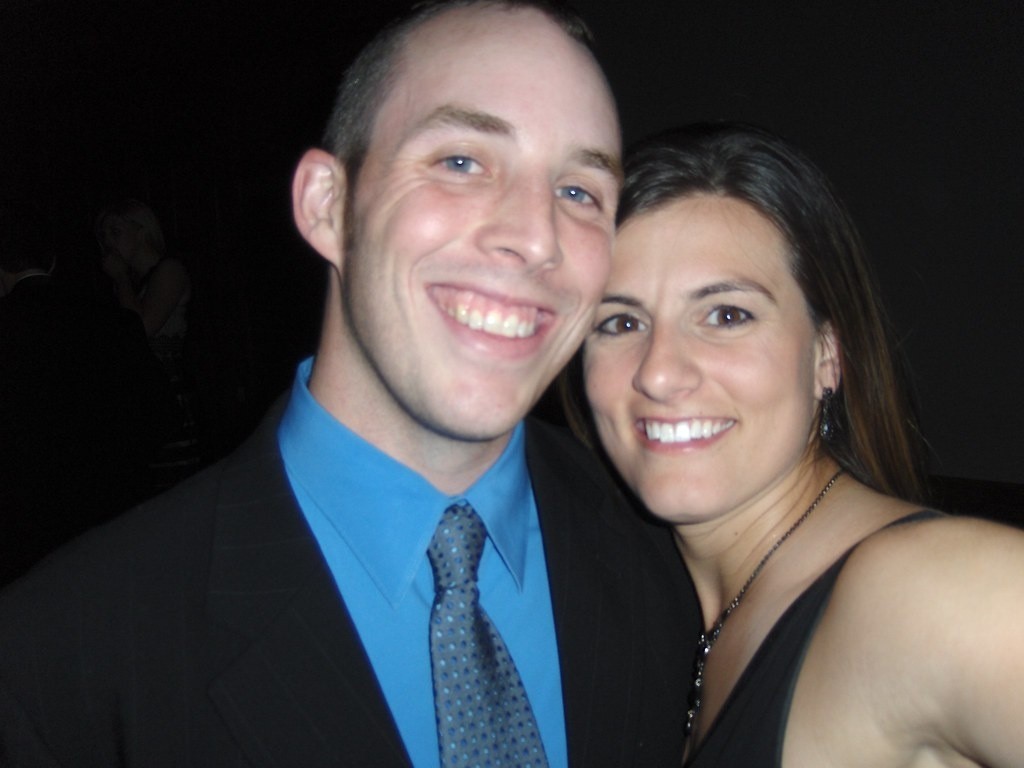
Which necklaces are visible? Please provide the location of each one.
[686,466,849,729]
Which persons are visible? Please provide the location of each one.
[559,131,1023,768]
[0,0,704,768]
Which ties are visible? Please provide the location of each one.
[425,502,549,768]
